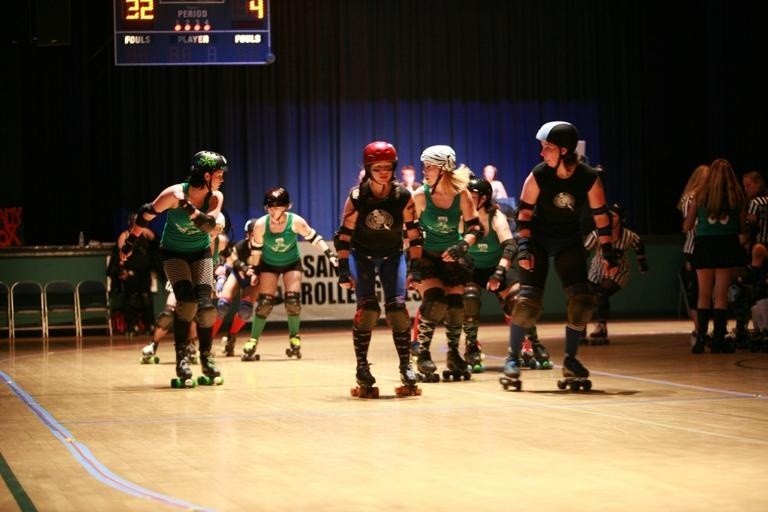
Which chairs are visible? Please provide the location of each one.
[675,270,699,322]
[0,273,156,344]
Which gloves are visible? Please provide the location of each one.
[636,256,648,272]
[486,266,505,284]
[337,258,353,288]
[448,239,470,261]
[517,238,531,261]
[122,234,135,260]
[601,242,616,268]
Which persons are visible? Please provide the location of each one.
[412,144,481,373]
[330,139,423,386]
[116,149,232,381]
[502,118,614,380]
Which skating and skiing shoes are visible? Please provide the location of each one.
[241,337,260,361]
[498,348,522,392]
[225,332,236,356]
[556,351,592,392]
[198,345,224,386]
[350,358,380,397]
[170,344,196,388]
[415,349,440,383]
[464,339,486,373]
[521,326,554,370]
[690,321,768,354]
[392,361,422,397]
[286,333,302,358]
[140,341,160,363]
[442,347,472,382]
[590,322,609,346]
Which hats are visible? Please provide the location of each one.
[190,149,229,171]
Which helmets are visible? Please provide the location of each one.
[362,140,398,166]
[467,179,492,195]
[536,120,578,152]
[263,186,289,208]
[420,144,457,173]
